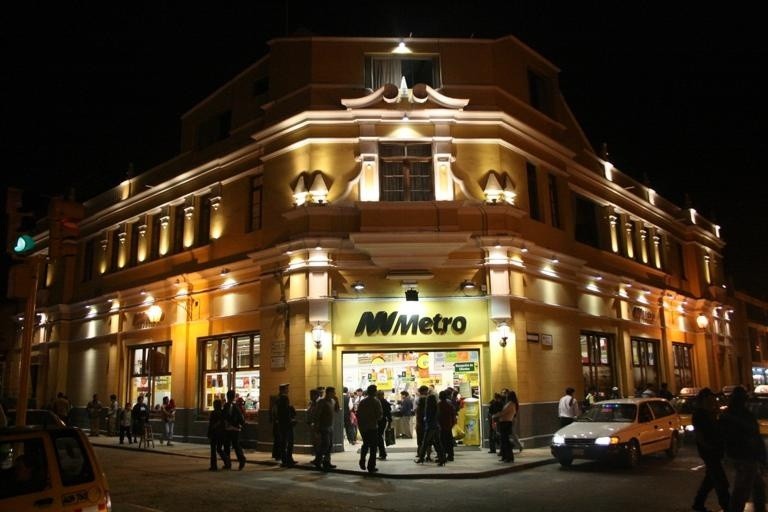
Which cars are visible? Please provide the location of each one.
[670,387,718,439]
[550,397,681,468]
[6,408,68,428]
[717,385,749,414]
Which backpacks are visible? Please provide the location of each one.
[230,402,245,424]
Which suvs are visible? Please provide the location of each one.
[0,427,112,512]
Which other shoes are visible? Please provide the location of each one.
[239,457,246,470]
[379,455,386,460]
[414,452,454,467]
[222,465,232,470]
[489,450,513,464]
[276,456,336,470]
[359,461,365,470]
[368,469,378,471]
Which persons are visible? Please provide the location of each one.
[642,382,673,400]
[557,385,619,427]
[208,390,252,470]
[343,384,478,472]
[3,391,17,409]
[107,394,177,446]
[12,454,39,495]
[692,386,768,511]
[306,386,340,468]
[269,384,298,464]
[488,388,522,461]
[86,393,103,436]
[41,392,69,423]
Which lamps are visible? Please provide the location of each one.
[388,38,415,58]
[695,312,709,331]
[493,319,512,349]
[310,318,327,362]
[462,279,474,292]
[350,279,365,292]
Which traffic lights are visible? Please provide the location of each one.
[7,189,35,257]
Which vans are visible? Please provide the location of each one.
[746,384,768,439]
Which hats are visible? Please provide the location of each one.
[279,384,290,389]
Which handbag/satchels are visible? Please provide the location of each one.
[385,428,395,446]
